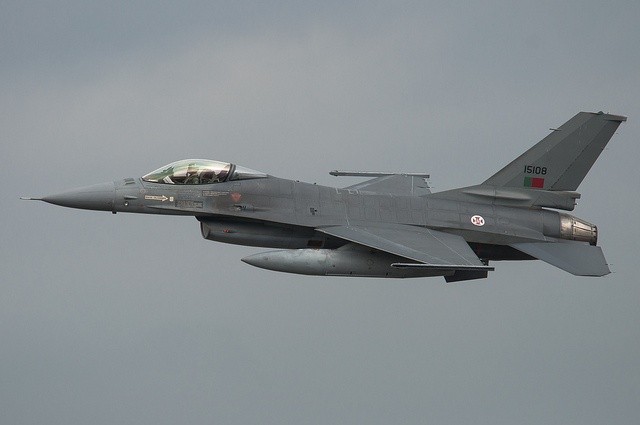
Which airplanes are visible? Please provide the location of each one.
[18,111,628,282]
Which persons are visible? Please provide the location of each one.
[184,163,199,184]
